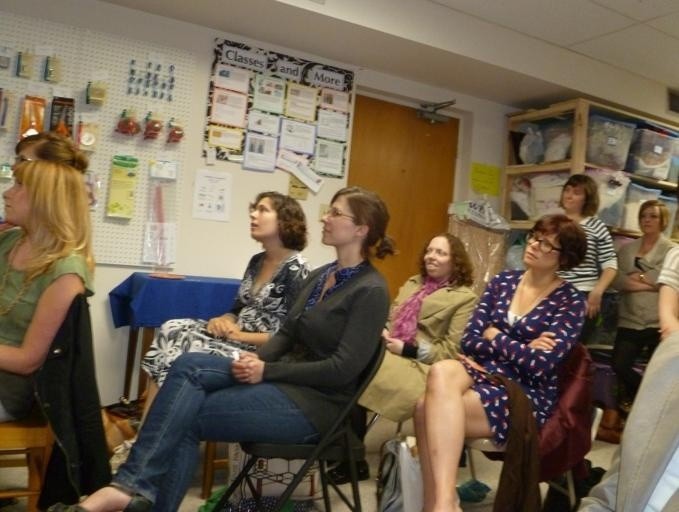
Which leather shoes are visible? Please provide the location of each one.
[320,456,374,488]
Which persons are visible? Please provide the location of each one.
[44,185,393,511]
[573,328,678,512]
[555,173,618,344]
[1,158,92,419]
[15,131,91,184]
[142,190,312,429]
[411,210,588,512]
[653,243,678,341]
[324,231,478,483]
[611,197,677,392]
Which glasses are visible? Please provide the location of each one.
[13,154,39,165]
[524,229,564,254]
[321,206,361,222]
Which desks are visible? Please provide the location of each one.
[109,271,243,414]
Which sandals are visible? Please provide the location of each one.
[45,481,154,512]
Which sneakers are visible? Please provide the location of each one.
[108,435,139,475]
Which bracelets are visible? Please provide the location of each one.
[638,272,645,282]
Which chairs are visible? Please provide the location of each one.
[361,412,577,512]
[502,98,679,291]
[0,294,88,512]
[201,334,387,512]
